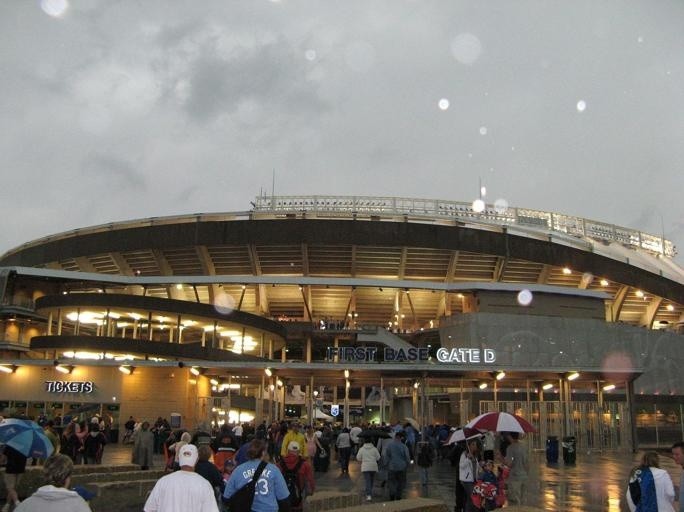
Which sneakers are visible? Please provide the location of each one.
[390,495,401,501]
[365,495,371,501]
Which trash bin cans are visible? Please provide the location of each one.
[562,436,576,466]
[546,436,559,463]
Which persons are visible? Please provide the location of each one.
[123,415,530,511]
[626,451,676,512]
[670,441,684,511]
[317,316,350,330]
[1,414,107,511]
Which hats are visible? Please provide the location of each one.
[179,444,198,467]
[288,441,299,451]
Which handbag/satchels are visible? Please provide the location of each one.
[226,481,255,512]
[350,439,354,448]
[320,448,328,458]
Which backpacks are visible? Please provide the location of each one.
[277,458,307,507]
